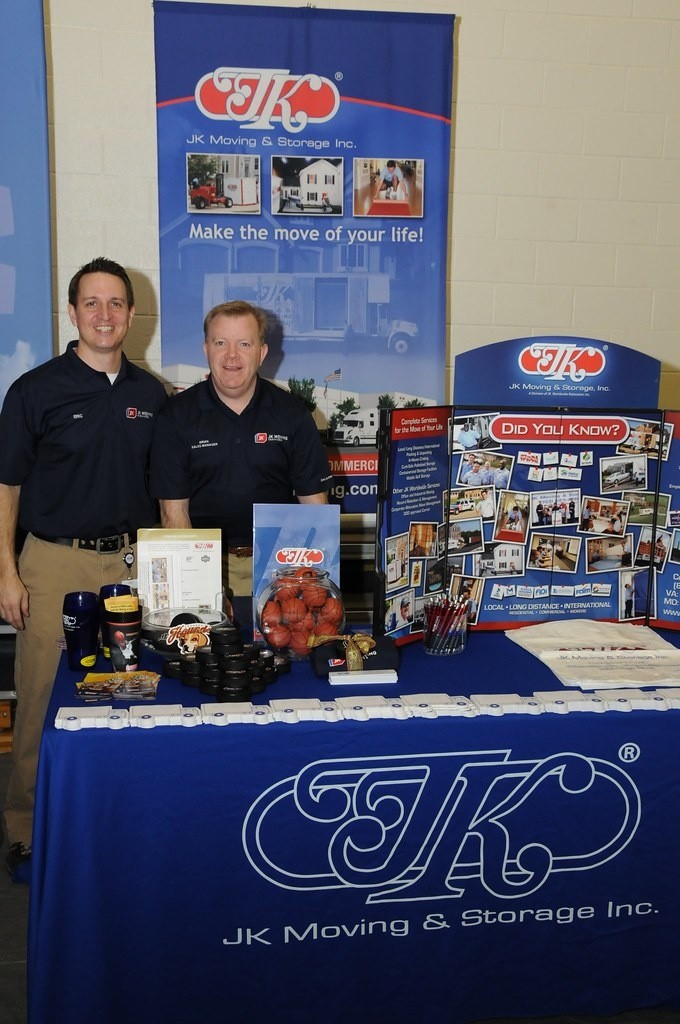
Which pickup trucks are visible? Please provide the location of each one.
[450,500,475,514]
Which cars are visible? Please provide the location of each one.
[491,569,511,575]
[633,472,645,484]
[605,472,630,484]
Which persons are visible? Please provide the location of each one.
[655,534,666,558]
[529,546,554,568]
[460,454,510,490]
[374,160,408,204]
[624,584,635,619]
[457,422,481,451]
[583,505,625,534]
[593,550,600,563]
[635,472,639,486]
[502,506,524,531]
[0,256,170,885]
[476,491,493,521]
[622,541,631,566]
[151,300,333,621]
[395,596,411,629]
[536,498,575,526]
[460,581,476,623]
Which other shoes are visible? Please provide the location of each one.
[5,842,31,876]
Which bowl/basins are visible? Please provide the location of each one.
[141,606,229,661]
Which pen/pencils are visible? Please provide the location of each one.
[424,592,470,655]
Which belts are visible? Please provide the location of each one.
[223,546,253,558]
[31,531,137,555]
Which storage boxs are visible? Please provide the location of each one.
[223,177,258,206]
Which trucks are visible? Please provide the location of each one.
[332,408,379,446]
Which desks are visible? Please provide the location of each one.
[544,552,570,571]
[43,625,680,1024]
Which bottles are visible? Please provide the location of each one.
[256,567,346,660]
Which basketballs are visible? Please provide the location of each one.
[261,567,344,656]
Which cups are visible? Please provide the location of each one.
[423,604,468,656]
[62,584,142,673]
